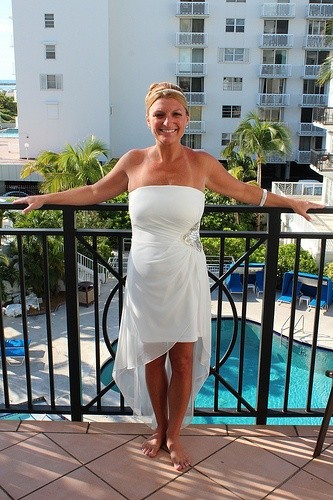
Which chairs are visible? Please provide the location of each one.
[277,281,303,305]
[309,285,328,310]
[255,271,264,297]
[0,339,31,366]
[229,273,243,296]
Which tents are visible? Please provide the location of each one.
[225,261,265,292]
[282,271,332,309]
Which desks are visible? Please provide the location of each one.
[25,297,40,311]
[248,284,255,294]
[5,304,26,318]
[298,296,310,308]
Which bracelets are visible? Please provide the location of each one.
[258,188,268,207]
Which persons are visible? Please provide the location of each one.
[12,82,326,470]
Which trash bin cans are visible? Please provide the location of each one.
[78,280,95,309]
